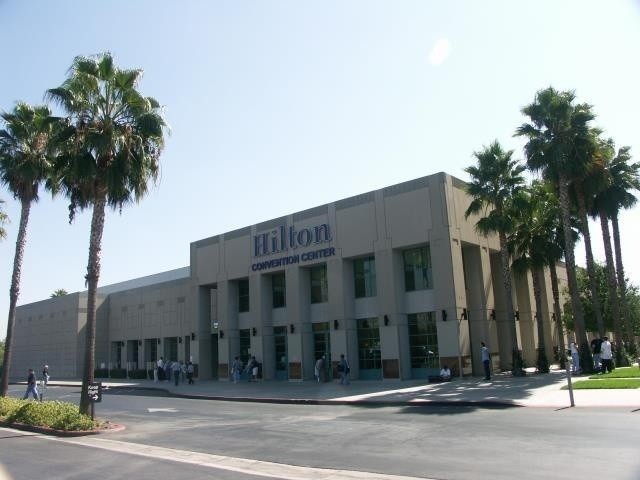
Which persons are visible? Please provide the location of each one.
[149,355,195,386]
[42,364,50,389]
[313,355,325,383]
[337,353,350,385]
[229,351,260,384]
[23,367,40,401]
[441,366,451,380]
[569,338,615,376]
[479,341,493,381]
